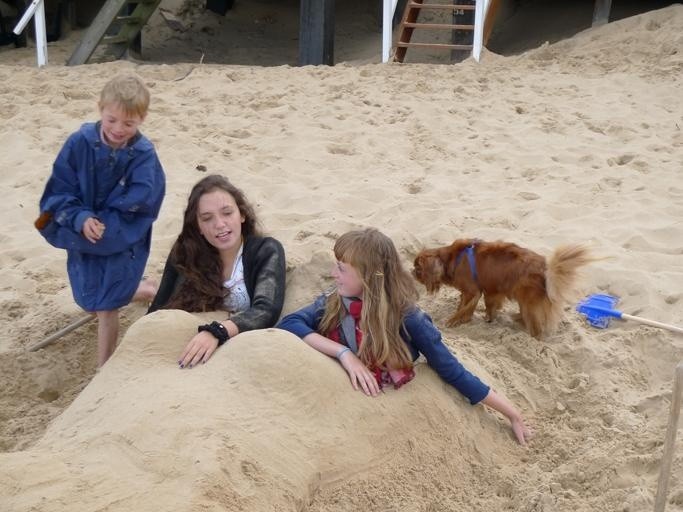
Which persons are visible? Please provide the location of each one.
[146,174,286,369]
[35,74,165,376]
[276,228,533,447]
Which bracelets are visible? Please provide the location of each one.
[336,347,350,359]
[197,321,230,347]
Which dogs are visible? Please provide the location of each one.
[411,238,616,341]
